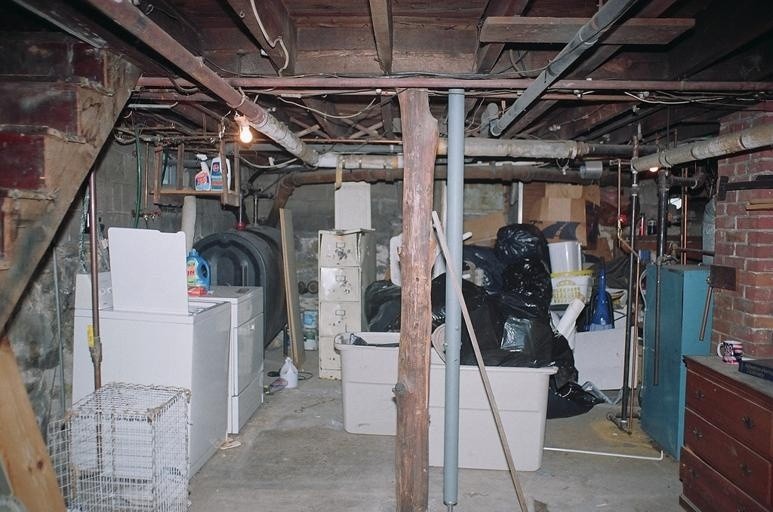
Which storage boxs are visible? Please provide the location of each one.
[328,327,560,474]
[545,239,595,309]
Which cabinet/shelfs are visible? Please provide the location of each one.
[195,283,268,439]
[316,227,376,382]
[677,351,772,510]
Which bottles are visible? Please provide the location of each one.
[639,212,646,237]
[647,218,657,235]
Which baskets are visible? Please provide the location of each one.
[548,269,594,304]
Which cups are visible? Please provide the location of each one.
[717,341,743,364]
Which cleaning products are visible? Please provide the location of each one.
[187,247,211,292]
[195,153,210,192]
[280,356,299,389]
[210,153,231,192]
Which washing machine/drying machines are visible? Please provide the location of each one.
[71,226,229,484]
[188,285,266,432]
